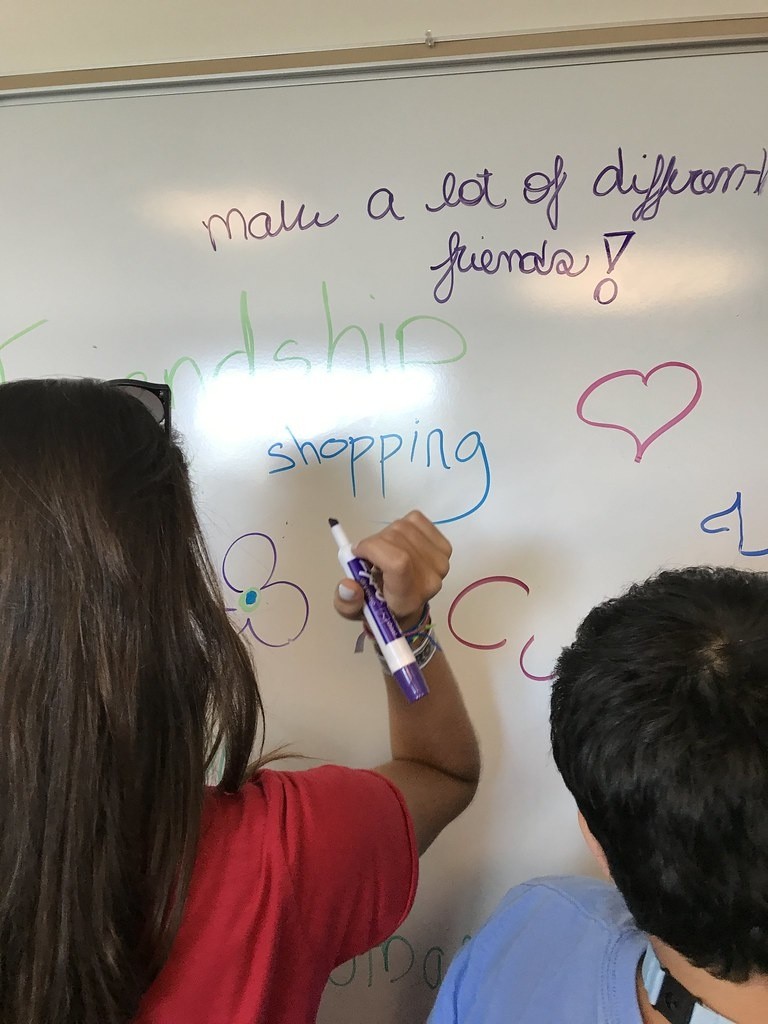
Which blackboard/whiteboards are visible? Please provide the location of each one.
[0,12,768,1024]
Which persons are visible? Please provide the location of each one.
[426,564,768,1024]
[1,379,482,1024]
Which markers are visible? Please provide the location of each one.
[328,517,430,703]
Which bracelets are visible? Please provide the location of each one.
[353,603,442,681]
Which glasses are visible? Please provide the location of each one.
[99,378,173,440]
